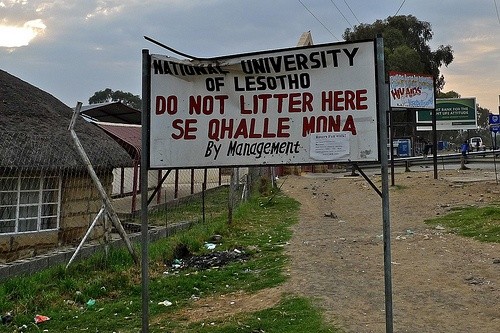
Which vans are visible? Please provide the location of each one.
[467,136,485,151]
